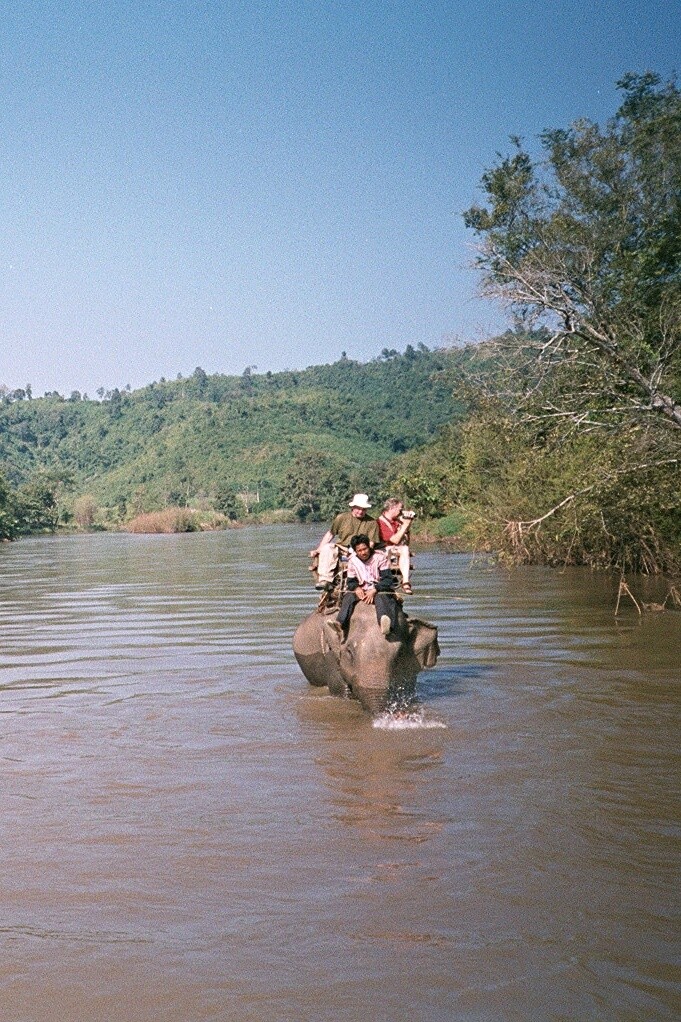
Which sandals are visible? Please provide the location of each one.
[401,580,413,595]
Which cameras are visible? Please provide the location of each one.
[401,511,416,518]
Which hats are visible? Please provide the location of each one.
[349,494,372,509]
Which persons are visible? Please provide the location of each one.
[327,534,396,634]
[376,498,413,595]
[311,494,380,591]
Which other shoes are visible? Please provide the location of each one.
[315,583,333,591]
[380,615,392,635]
[327,619,341,633]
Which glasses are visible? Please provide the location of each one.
[397,507,405,512]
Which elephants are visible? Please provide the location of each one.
[293,597,441,714]
